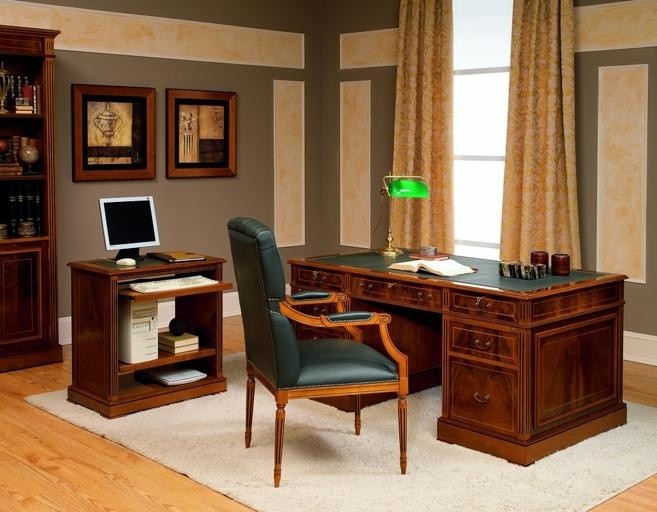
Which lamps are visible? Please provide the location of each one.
[378,173,429,257]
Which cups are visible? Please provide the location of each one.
[530,251,548,273]
[551,253,570,276]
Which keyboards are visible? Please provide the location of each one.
[129,275,218,293]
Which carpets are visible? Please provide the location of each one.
[22,350,657,511]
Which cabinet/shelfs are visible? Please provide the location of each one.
[1,25,63,373]
[66,251,234,416]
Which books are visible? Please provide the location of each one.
[142,368,208,386]
[158,332,199,348]
[387,259,476,277]
[146,251,207,263]
[0,135,41,177]
[158,343,199,353]
[9,73,43,115]
[409,253,449,261]
[4,193,44,238]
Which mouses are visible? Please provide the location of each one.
[116,258,136,266]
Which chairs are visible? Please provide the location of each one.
[228,216,408,486]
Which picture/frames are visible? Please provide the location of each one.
[70,84,156,181]
[165,87,237,179]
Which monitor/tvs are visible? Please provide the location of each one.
[98,195,161,262]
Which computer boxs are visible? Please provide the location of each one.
[119,296,158,364]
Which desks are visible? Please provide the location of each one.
[288,247,629,467]
